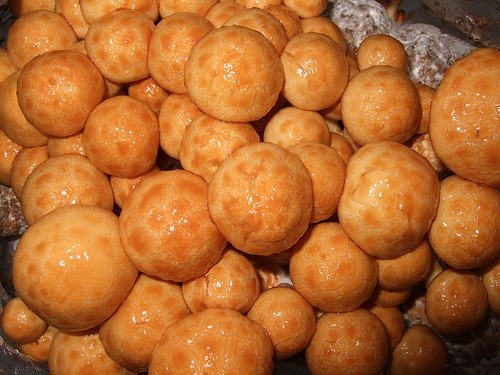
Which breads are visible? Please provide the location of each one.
[0,0,500,375]
